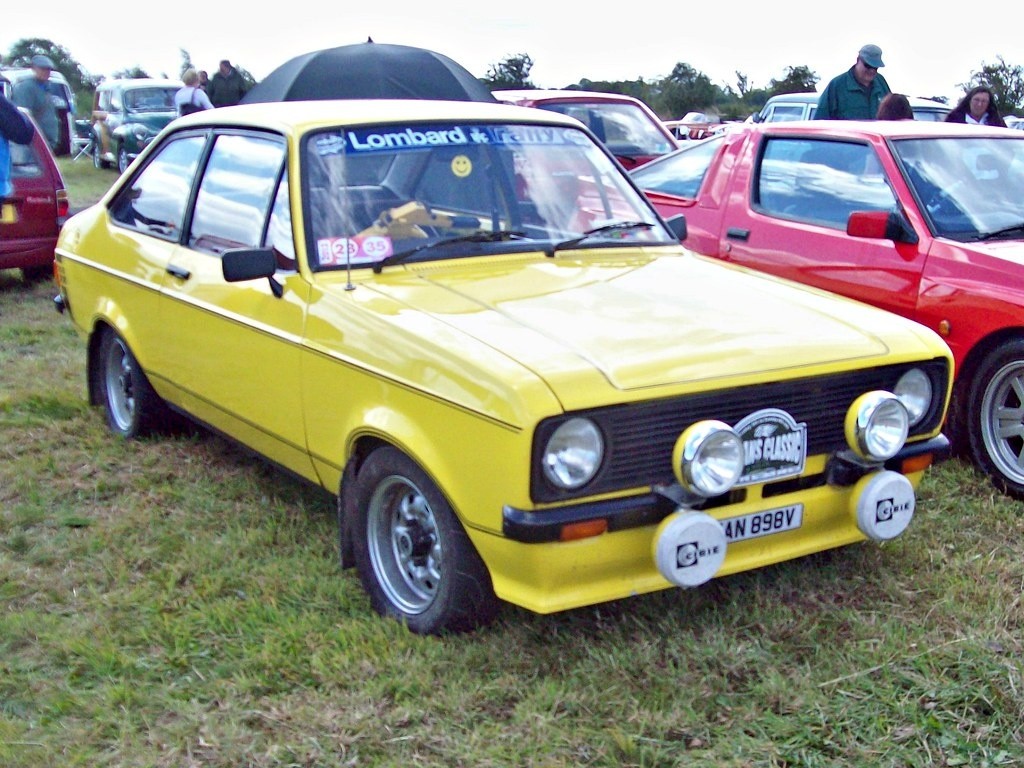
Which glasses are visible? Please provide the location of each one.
[862,59,878,69]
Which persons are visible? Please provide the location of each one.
[813,44,914,121]
[12,54,59,156]
[175,60,249,118]
[943,85,1008,127]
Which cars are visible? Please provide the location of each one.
[569,120,1023,506]
[491,90,681,203]
[47,366,52,367]
[89,78,186,174]
[49,100,953,632]
[748,92,956,123]
[0,106,70,280]
[663,120,743,141]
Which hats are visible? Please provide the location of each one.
[32,55,53,67]
[859,44,884,67]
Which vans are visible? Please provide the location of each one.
[0,66,78,157]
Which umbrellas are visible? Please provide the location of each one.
[238,35,499,104]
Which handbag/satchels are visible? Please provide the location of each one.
[180,88,205,116]
